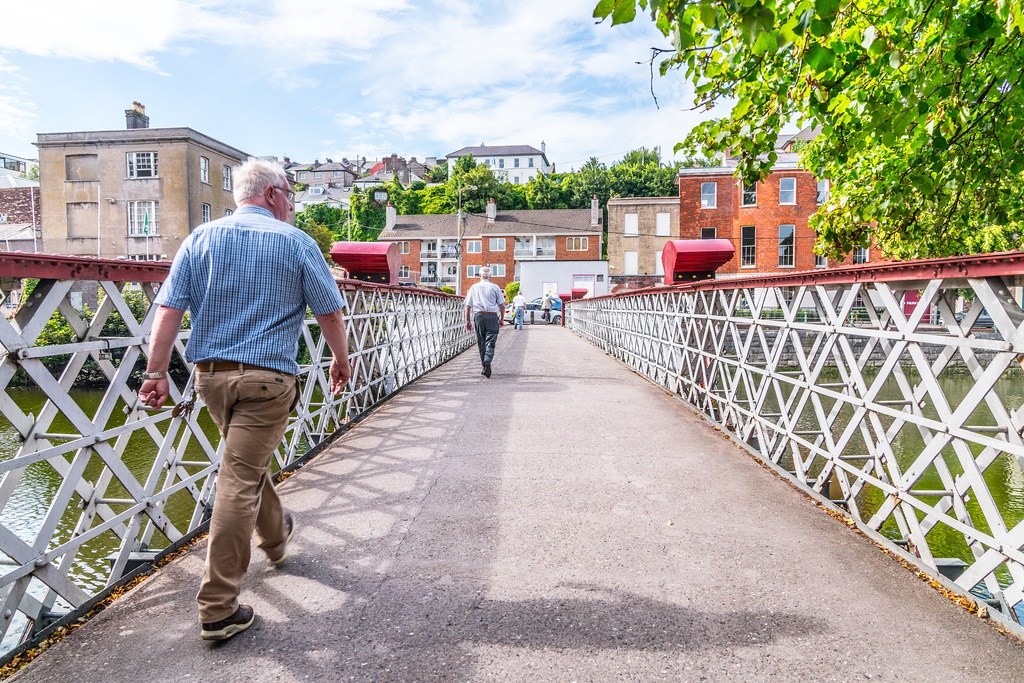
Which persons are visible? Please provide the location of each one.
[541,293,553,322]
[463,265,506,378]
[512,291,527,330]
[137,156,351,639]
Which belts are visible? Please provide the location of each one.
[478,311,497,314]
[195,362,267,372]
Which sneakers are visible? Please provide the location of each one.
[269,513,295,564]
[201,604,254,640]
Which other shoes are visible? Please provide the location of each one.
[480,370,485,375]
[515,323,517,329]
[485,361,491,378]
[520,328,522,329]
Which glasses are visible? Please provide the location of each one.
[273,186,295,201]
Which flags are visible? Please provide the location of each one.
[370,163,385,174]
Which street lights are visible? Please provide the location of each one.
[327,196,353,244]
[13,174,38,254]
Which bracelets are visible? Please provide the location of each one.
[466,320,471,324]
[142,370,168,379]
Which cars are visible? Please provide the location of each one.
[938,306,994,329]
[499,302,562,325]
[528,296,562,311]
